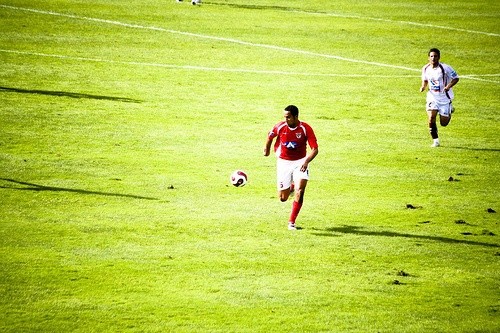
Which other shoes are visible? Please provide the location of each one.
[451,104,455,112]
[432,142,440,147]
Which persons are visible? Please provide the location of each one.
[420,48,459,147]
[264,105,318,230]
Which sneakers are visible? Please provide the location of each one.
[288,222,296,230]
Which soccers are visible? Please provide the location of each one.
[228,170,249,188]
